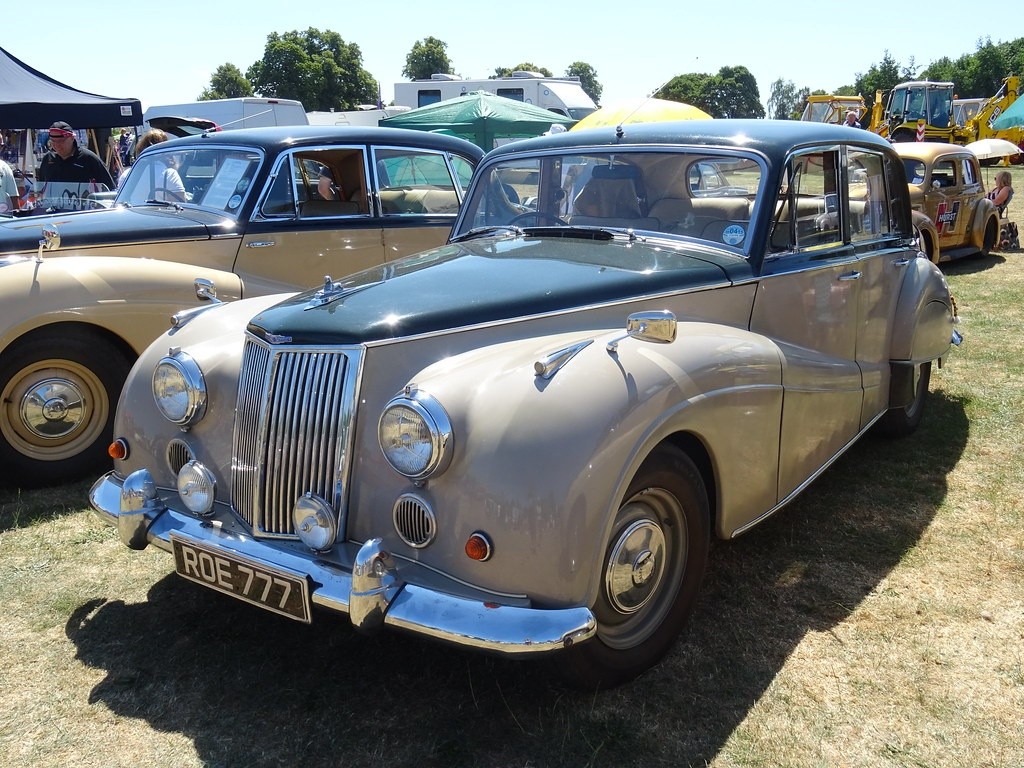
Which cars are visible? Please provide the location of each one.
[525,158,753,199]
[90,118,963,678]
[848,140,1001,264]
[0,123,540,485]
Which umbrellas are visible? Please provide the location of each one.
[990,93,1024,130]
[568,98,713,166]
[965,138,1024,185]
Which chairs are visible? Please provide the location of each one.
[998,192,1014,225]
[701,221,748,248]
[570,216,660,231]
[302,201,360,216]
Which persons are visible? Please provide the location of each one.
[117,128,185,205]
[986,171,1012,206]
[843,106,861,129]
[0,133,30,209]
[27,121,115,214]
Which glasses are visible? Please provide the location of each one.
[995,177,996,180]
[49,135,70,142]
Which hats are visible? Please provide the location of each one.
[49,121,73,134]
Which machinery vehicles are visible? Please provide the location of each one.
[798,95,867,138]
[867,68,1024,166]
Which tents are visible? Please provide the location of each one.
[0,47,143,169]
[376,90,581,185]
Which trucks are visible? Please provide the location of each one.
[393,69,600,121]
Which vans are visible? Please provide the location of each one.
[127,97,311,176]
